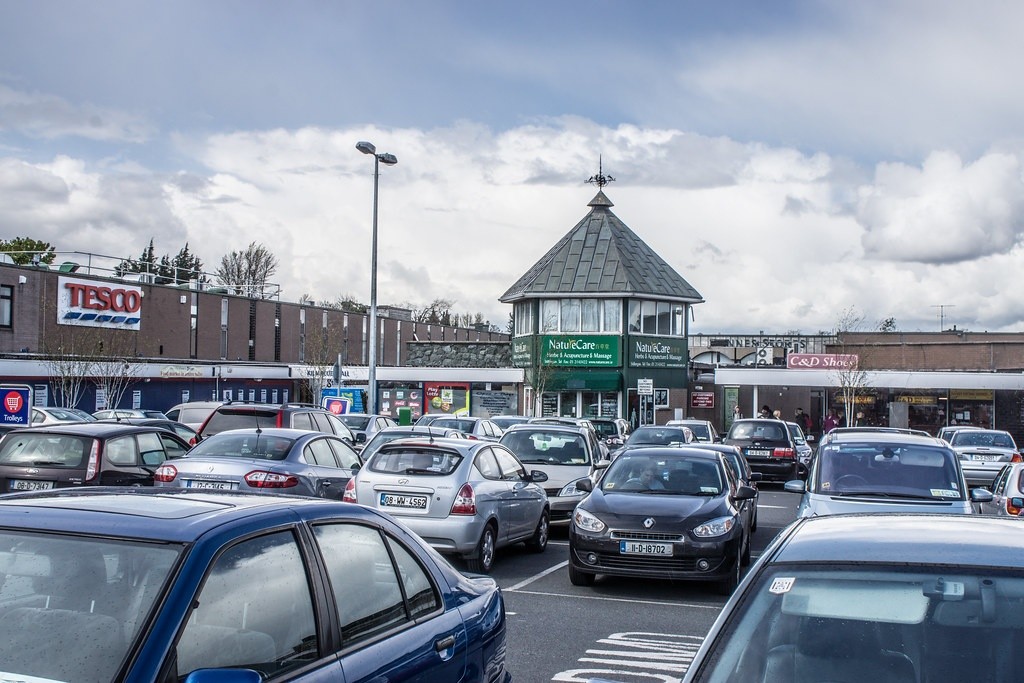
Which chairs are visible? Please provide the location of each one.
[764,617,916,683]
[0,541,121,683]
[175,556,280,677]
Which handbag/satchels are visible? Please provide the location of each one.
[822,421,826,429]
[805,416,813,428]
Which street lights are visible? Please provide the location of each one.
[355,141,398,412]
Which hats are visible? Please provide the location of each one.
[762,405,769,411]
[797,408,803,410]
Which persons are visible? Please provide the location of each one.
[622,460,665,489]
[757,405,780,419]
[982,421,990,429]
[824,409,846,434]
[795,408,812,440]
[732,407,743,420]
[855,411,868,427]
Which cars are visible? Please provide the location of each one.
[0,485,514,683]
[785,421,815,469]
[30,396,732,469]
[585,512,1024,683]
[0,422,193,494]
[948,429,1024,490]
[154,427,365,501]
[569,446,758,597]
[936,426,986,444]
[979,449,1024,518]
[342,437,551,575]
[496,423,613,532]
[720,419,800,490]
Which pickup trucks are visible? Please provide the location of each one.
[783,427,994,515]
